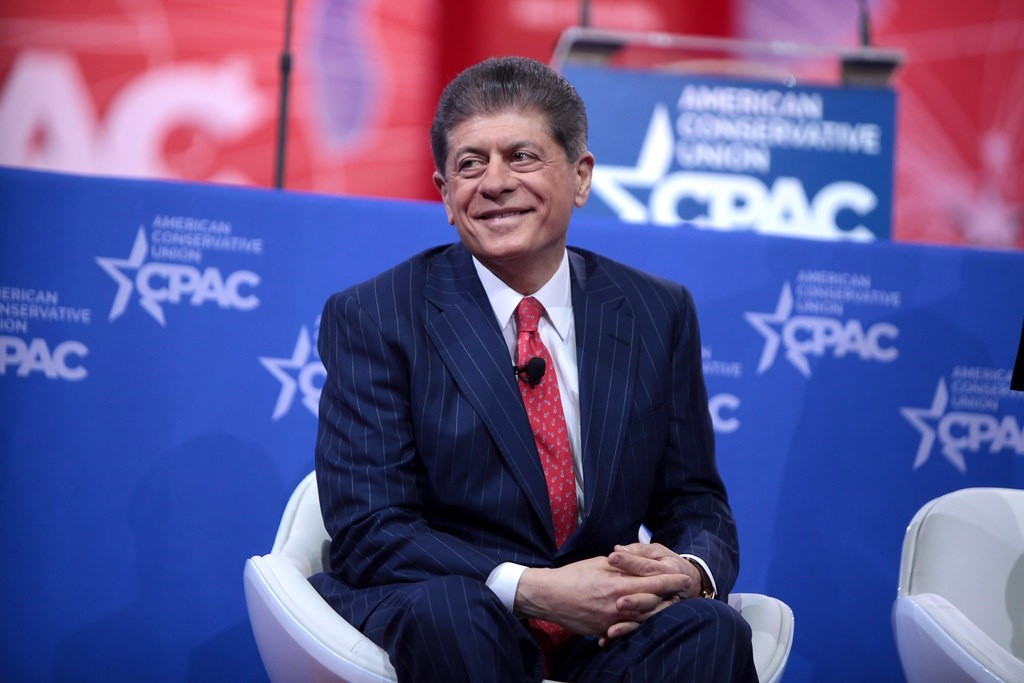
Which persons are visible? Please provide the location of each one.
[305,55,763,682]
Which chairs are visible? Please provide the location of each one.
[245,469,794,682]
[893,488,1023,683]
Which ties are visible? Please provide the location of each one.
[511,295,582,676]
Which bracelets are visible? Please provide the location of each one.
[682,555,718,600]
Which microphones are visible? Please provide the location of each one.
[527,357,546,388]
